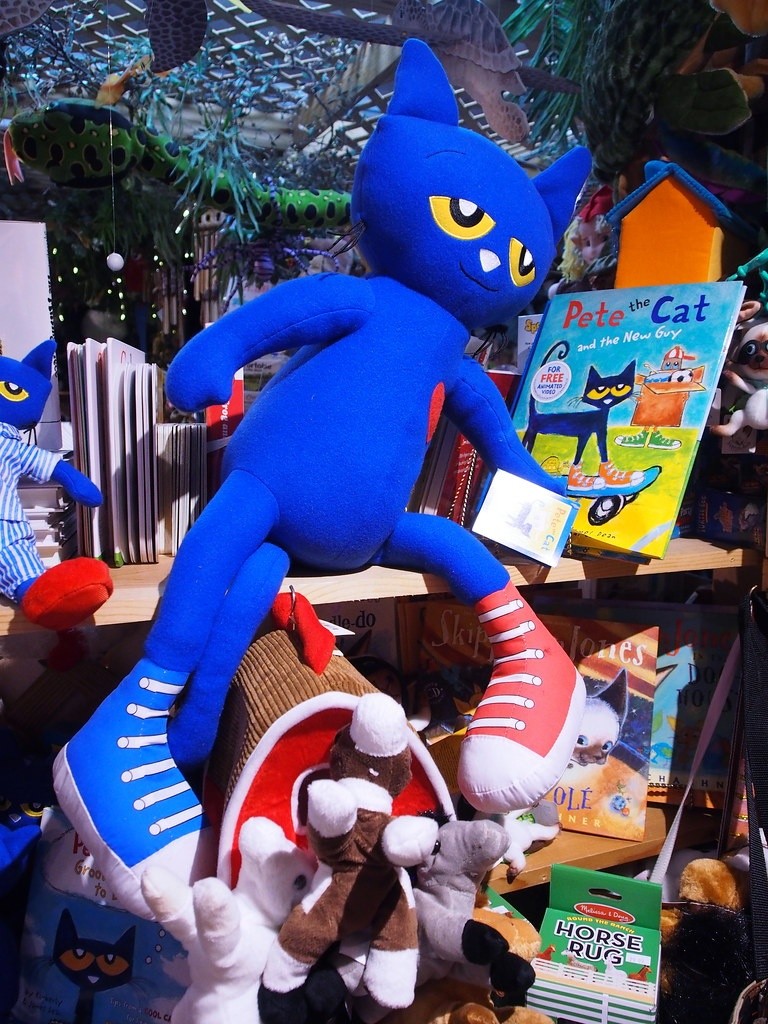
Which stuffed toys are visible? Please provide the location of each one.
[53,39,595,925]
[0,340,114,625]
[605,0,768,297]
[0,587,561,1024]
[625,835,768,1024]
[710,301,768,437]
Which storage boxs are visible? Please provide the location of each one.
[524,863,662,1024]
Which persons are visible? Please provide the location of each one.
[548,185,613,297]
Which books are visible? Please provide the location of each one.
[410,280,748,567]
[17,335,208,568]
[317,585,737,841]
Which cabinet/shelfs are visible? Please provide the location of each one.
[0,535,763,897]
